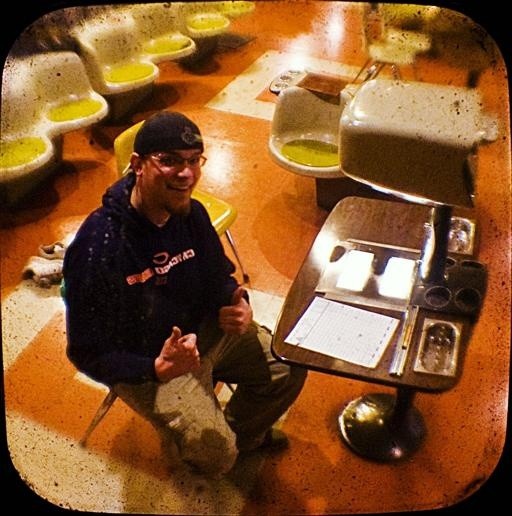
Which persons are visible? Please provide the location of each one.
[62,110,308,481]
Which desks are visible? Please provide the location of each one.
[272,197,483,464]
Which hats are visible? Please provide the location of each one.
[116,110,205,175]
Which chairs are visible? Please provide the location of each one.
[0,52,107,204]
[353,2,417,83]
[168,2,255,65]
[269,87,349,177]
[114,121,248,285]
[68,4,195,118]
[371,3,431,81]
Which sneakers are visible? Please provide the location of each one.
[38,241,67,259]
[22,256,64,287]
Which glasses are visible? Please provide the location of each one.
[148,153,207,168]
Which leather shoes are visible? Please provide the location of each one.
[261,428,287,450]
